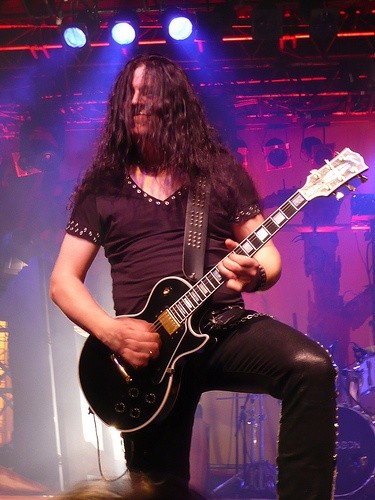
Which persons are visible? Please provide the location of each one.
[48,54,340,500]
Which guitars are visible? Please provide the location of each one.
[74,144,369,437]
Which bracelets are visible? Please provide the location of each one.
[251,262,266,292]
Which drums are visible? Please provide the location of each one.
[358,352,375,416]
[332,402,375,498]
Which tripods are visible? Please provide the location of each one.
[210,394,279,496]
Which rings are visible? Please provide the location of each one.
[149,348,153,357]
